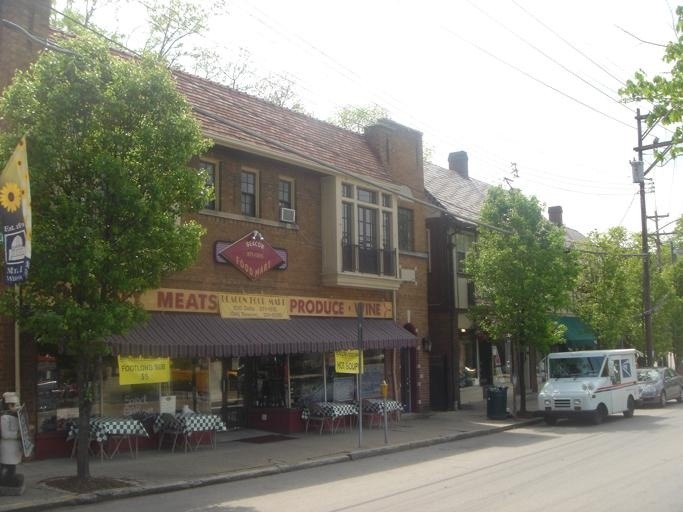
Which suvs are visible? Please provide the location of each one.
[36,381,81,411]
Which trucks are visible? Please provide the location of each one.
[535,348,642,426]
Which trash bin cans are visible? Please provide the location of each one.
[487,386,508,420]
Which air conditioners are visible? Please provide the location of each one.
[278,207,296,223]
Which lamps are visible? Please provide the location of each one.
[422,337,432,354]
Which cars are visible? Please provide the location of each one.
[164,360,238,393]
[635,367,682,406]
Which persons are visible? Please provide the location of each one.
[0,392,24,485]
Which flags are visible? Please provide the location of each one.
[0,138,37,284]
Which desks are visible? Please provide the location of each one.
[301,401,354,440]
[67,415,148,464]
[359,397,402,431]
[152,411,224,455]
